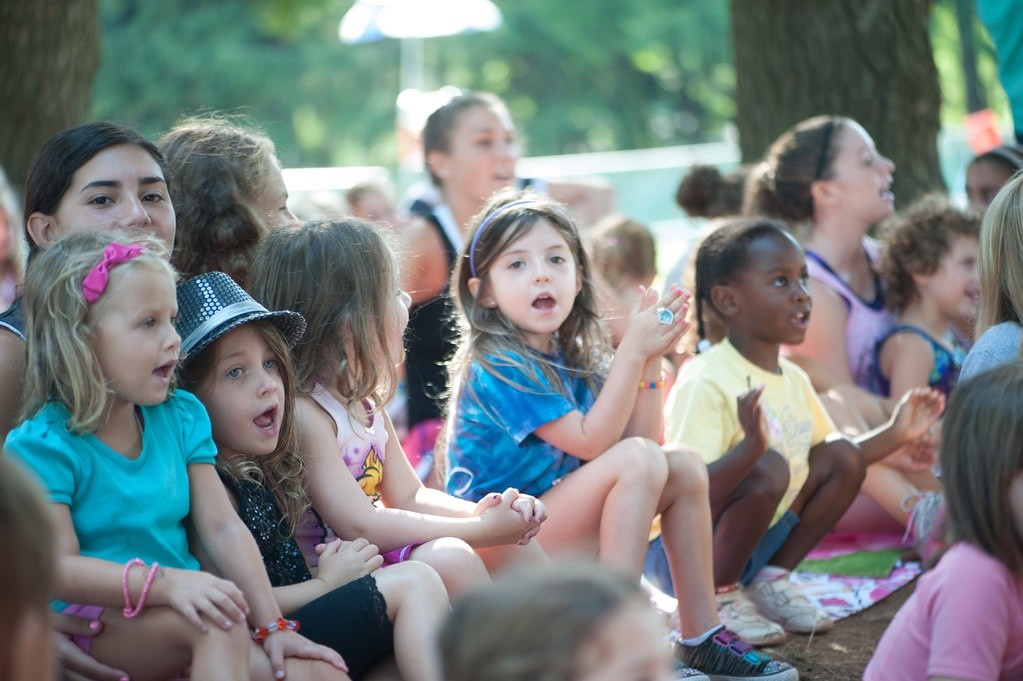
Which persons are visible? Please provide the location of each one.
[0,90,1023,681]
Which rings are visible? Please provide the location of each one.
[657,308,673,325]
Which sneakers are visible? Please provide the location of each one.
[670,657,710,681]
[743,573,834,634]
[714,582,786,644]
[672,624,799,681]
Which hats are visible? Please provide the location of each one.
[175,270,307,374]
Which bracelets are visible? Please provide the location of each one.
[122,558,160,618]
[639,381,665,388]
[253,618,300,643]
